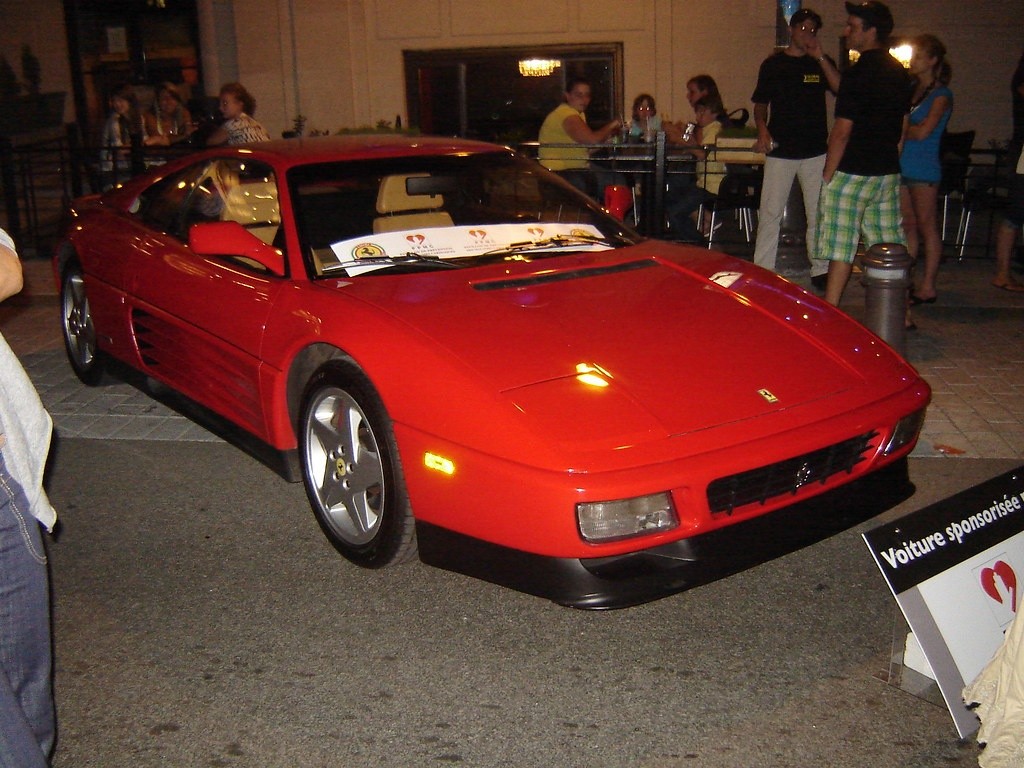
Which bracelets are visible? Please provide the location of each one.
[816,54,826,64]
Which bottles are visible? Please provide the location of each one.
[752,143,778,152]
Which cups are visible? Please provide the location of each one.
[619,128,628,144]
[645,128,656,142]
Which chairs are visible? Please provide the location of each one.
[938,129,976,241]
[955,152,1012,264]
[373,173,455,235]
[220,182,324,274]
[537,165,766,250]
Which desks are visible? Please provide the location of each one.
[596,139,698,234]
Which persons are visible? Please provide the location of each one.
[991,55,1024,293]
[537,75,737,230]
[749,8,842,292]
[204,83,272,148]
[812,0,919,332]
[99,81,193,171]
[0,221,60,768]
[896,32,955,308]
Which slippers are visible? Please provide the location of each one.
[992,281,1024,292]
[910,290,937,305]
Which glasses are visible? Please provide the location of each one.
[801,26,816,34]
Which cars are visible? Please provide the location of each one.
[54,131,932,607]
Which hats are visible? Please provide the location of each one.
[845,1,893,32]
[790,8,823,29]
